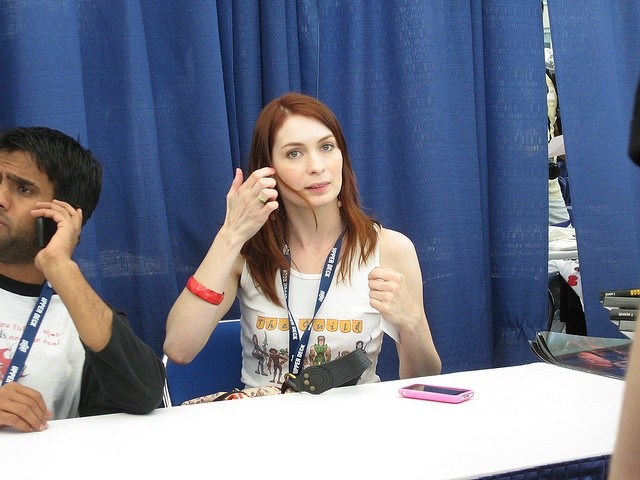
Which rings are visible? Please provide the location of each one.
[257,191,270,203]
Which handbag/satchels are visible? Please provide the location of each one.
[180,348,373,405]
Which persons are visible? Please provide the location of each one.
[0,126,165,431]
[335,350,349,360]
[251,334,268,376]
[355,341,366,380]
[268,348,288,384]
[303,355,308,371]
[309,336,331,367]
[606,311,640,480]
[163,92,442,406]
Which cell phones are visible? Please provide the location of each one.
[399,383,474,404]
[37,217,81,249]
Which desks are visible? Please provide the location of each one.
[0,361,627,476]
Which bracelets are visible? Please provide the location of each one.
[185,275,225,305]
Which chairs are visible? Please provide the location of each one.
[162,319,242,407]
